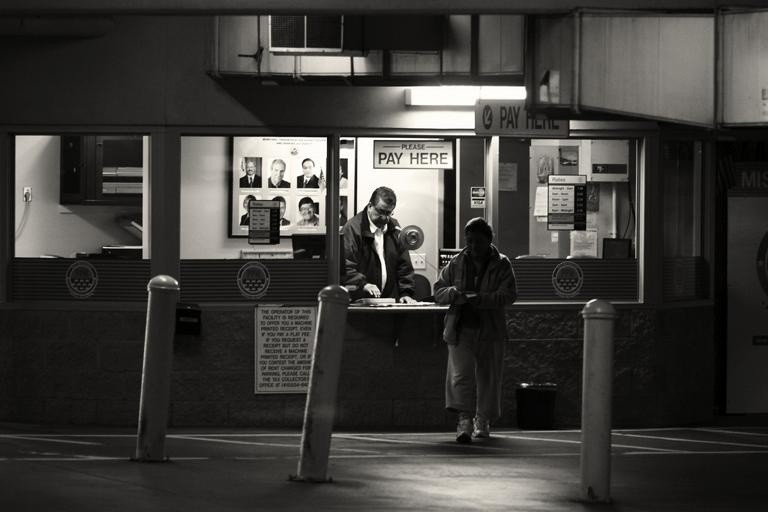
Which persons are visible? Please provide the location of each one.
[271,196,290,225]
[339,196,347,228]
[433,217,518,443]
[338,185,419,305]
[239,194,258,226]
[339,163,348,188]
[267,158,290,189]
[297,159,320,188]
[296,196,319,226]
[238,157,261,187]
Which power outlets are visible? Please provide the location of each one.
[24,187,31,202]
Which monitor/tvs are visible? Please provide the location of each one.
[292,233,347,278]
[602,238,632,259]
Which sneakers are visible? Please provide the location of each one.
[455,416,473,443]
[472,415,490,439]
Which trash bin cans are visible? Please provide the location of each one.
[515,383,558,430]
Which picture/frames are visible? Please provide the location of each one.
[228,136,357,239]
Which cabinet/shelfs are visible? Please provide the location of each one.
[61,135,144,206]
